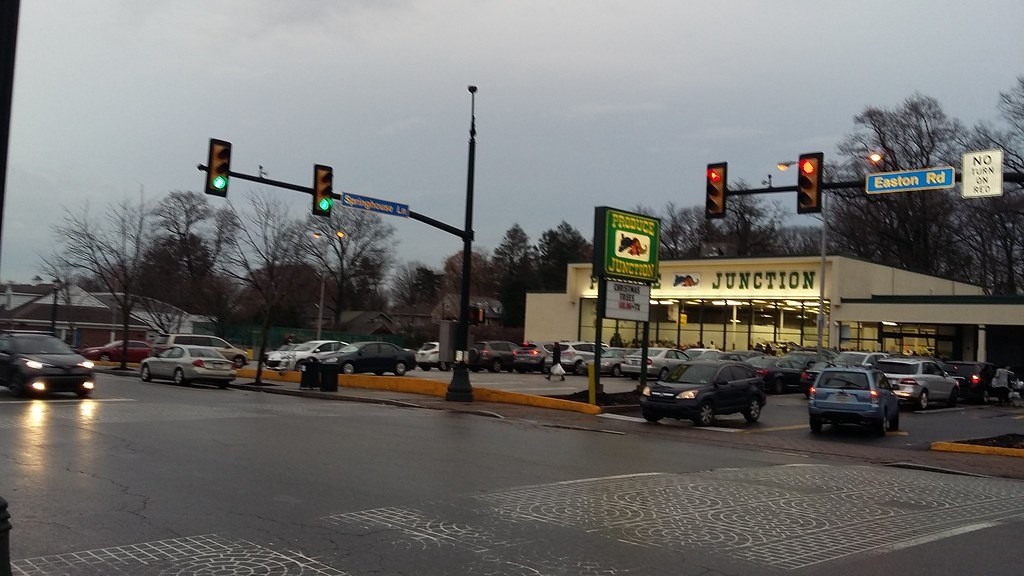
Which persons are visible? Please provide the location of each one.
[283,334,295,344]
[912,351,919,356]
[697,342,704,348]
[994,363,1015,407]
[750,342,778,356]
[709,340,716,349]
[544,342,565,381]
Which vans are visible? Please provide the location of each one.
[151,332,250,369]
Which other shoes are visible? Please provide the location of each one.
[560,378,565,381]
[545,376,550,381]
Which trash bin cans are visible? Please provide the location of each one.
[319,363,339,392]
[300,357,319,388]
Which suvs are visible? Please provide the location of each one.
[512,341,554,375]
[542,341,606,376]
[468,341,521,373]
[944,360,999,405]
[415,342,450,372]
[871,355,961,411]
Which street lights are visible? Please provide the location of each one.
[776,152,881,357]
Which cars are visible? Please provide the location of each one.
[785,346,891,399]
[264,340,359,373]
[684,348,768,362]
[808,367,899,439]
[317,340,416,374]
[620,347,693,382]
[582,348,640,378]
[262,343,300,364]
[80,339,151,363]
[139,344,238,389]
[638,358,768,428]
[745,354,811,395]
[0,329,95,397]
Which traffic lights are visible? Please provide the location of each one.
[313,163,333,217]
[705,161,728,219]
[797,152,824,215]
[203,137,232,199]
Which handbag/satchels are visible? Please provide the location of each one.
[992,378,997,388]
[550,363,566,376]
